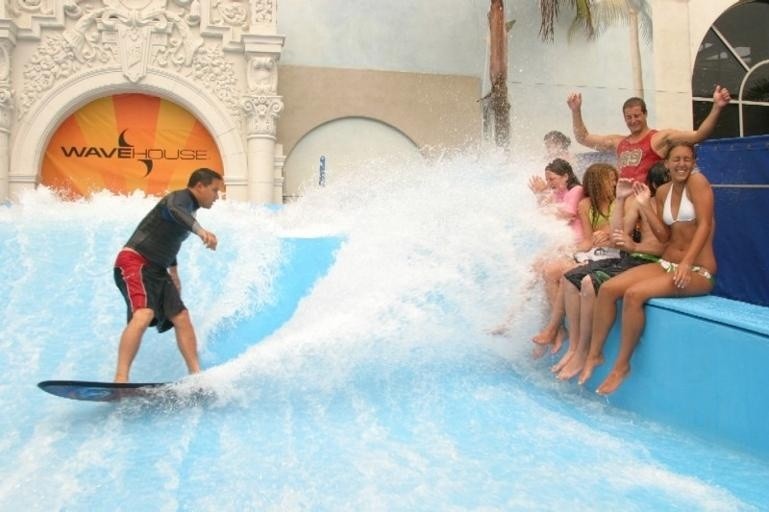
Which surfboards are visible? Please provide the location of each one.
[38,380,167,404]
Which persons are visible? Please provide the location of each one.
[548,158,677,381]
[575,139,719,395]
[487,157,588,354]
[541,129,575,162]
[565,83,733,190]
[527,163,620,361]
[110,167,226,383]
